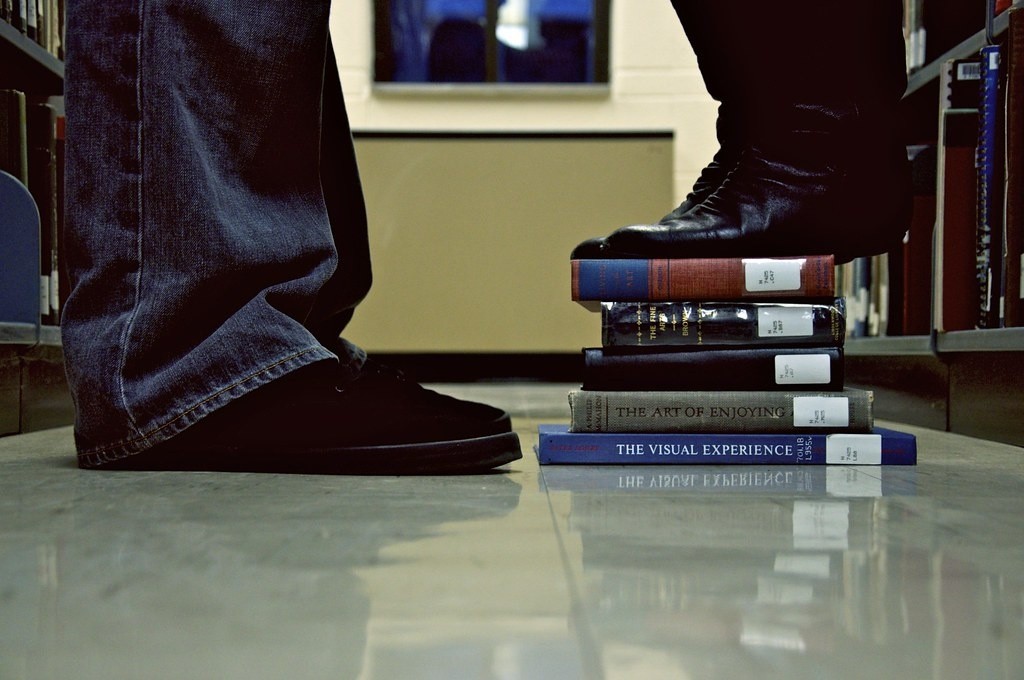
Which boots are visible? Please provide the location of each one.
[571,0,745,258]
[598,0,909,263]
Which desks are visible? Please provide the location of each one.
[338,130,674,383]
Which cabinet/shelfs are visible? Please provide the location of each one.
[0,0,85,434]
[831,0,1024,447]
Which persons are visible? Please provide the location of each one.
[55,476,523,680]
[571,1,910,260]
[61,0,524,475]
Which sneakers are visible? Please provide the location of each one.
[97,358,522,476]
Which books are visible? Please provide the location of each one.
[0,0,64,325]
[534,258,916,465]
[543,464,917,654]
[835,0,1024,337]
